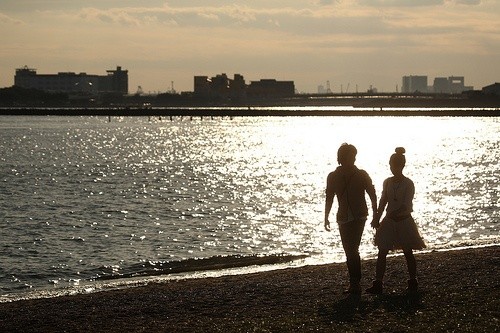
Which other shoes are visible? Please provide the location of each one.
[343,287,361,294]
[405,279,420,292]
[366,280,384,292]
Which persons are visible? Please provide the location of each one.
[366,146,426,292]
[324,143,378,294]
